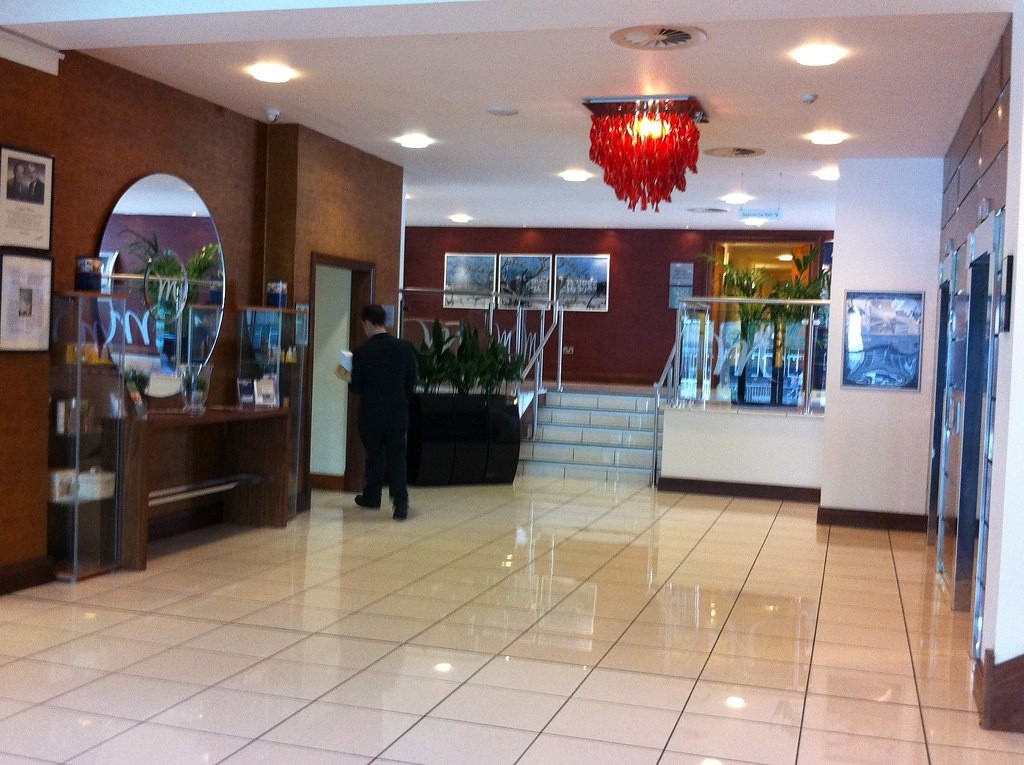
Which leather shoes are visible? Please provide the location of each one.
[354,494,381,509]
[393,494,408,520]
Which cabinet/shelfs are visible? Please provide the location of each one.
[53,288,309,583]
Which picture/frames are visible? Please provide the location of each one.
[553,254,610,312]
[839,287,925,393]
[443,253,496,310]
[498,253,552,311]
[0,146,55,251]
[0,253,55,355]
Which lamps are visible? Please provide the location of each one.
[738,171,783,226]
[582,95,708,213]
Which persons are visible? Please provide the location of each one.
[347,303,417,521]
[192,311,222,360]
[6,161,44,204]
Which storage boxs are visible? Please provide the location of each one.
[79,467,115,499]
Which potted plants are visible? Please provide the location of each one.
[762,245,825,406]
[124,224,221,394]
[706,251,767,404]
[407,317,521,489]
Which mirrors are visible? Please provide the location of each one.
[99,171,226,396]
